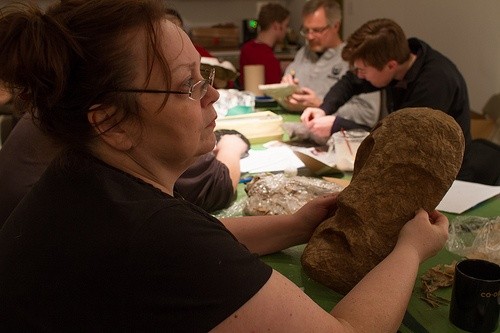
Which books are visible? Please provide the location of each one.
[210,82,308,145]
[199,57,240,89]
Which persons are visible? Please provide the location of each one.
[0,101,248,227]
[239,4,291,97]
[161,7,235,90]
[279,0,349,109]
[300,18,472,160]
[0,0,452,333]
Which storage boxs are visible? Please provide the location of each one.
[190,26,240,50]
[214,109,285,140]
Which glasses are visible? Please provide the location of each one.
[105,68,216,102]
[297,24,331,37]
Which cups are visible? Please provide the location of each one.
[449,258,500,333]
[332,130,370,172]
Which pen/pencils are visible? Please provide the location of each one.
[291,70,295,79]
[238,170,285,183]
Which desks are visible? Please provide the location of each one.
[208,107,500,333]
[199,49,296,89]
